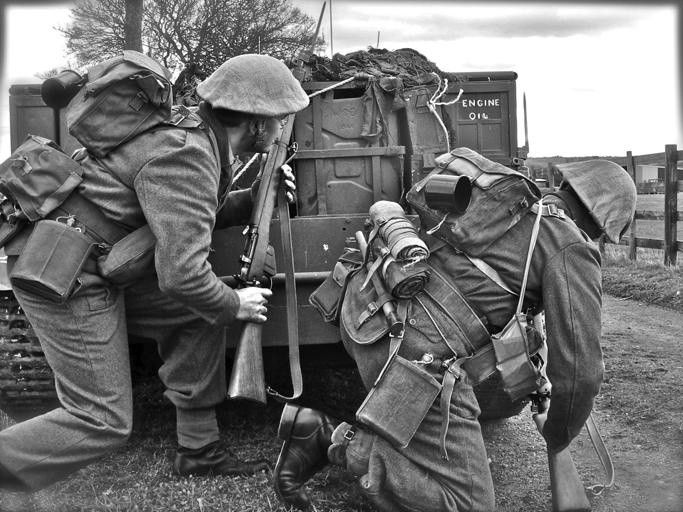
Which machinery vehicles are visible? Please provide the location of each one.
[0,73,519,411]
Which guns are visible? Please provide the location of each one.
[227,1,327,407]
[517,93,590,510]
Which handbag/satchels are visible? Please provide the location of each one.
[1,134,84,221]
[458,311,548,422]
[308,248,363,327]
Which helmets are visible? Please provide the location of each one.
[553,159,638,245]
[196,54,311,117]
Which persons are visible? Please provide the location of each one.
[1,52,311,497]
[274,157,640,512]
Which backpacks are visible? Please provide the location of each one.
[406,146,583,257]
[65,49,221,180]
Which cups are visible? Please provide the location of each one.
[38,68,86,112]
[424,174,473,217]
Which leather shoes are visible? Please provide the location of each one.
[274,401,343,511]
[175,441,272,478]
